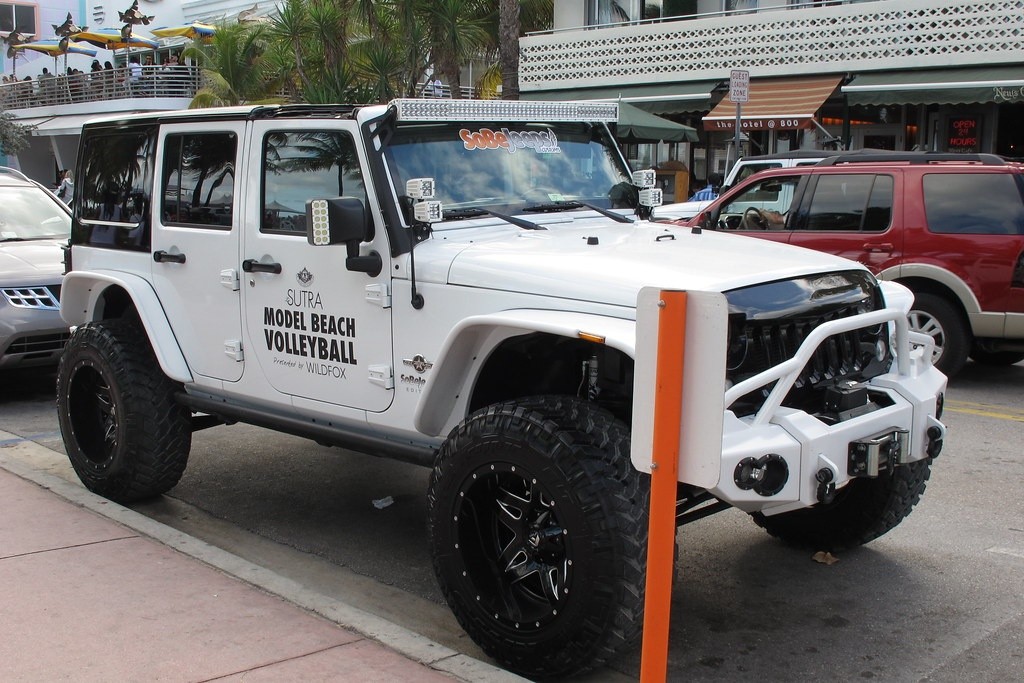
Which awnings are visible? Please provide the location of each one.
[701,77,845,131]
[518,81,721,115]
[840,63,1024,106]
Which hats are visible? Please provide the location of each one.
[102,182,119,196]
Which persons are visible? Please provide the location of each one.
[59,169,74,208]
[0,52,197,108]
[761,209,789,225]
[687,172,721,202]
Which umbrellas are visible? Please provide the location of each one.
[151,20,230,96]
[69,26,159,97]
[10,38,98,102]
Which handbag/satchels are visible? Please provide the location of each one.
[57,180,66,197]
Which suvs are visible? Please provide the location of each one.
[0,167,73,402]
[651,147,874,219]
[664,152,1024,380]
[57,99,947,683]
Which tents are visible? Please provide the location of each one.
[614,99,698,188]
